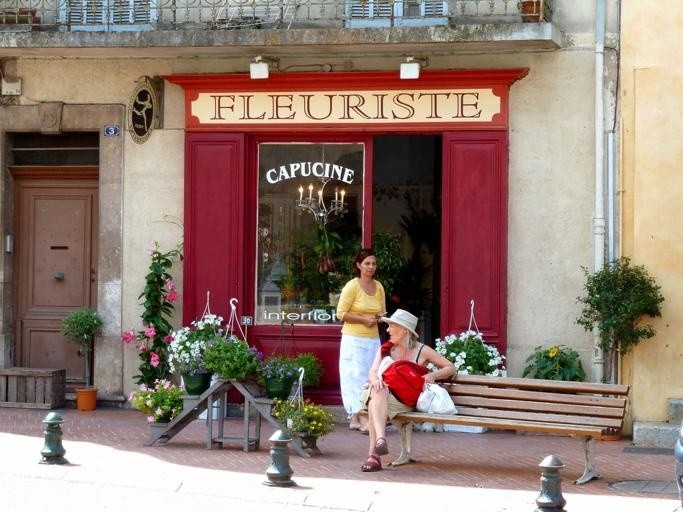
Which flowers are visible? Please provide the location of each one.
[127,378,187,423]
[519,344,587,394]
[189,328,267,380]
[424,330,508,378]
[179,351,210,379]
[166,313,224,375]
[258,353,325,389]
[271,397,336,437]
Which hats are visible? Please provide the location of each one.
[379,307,421,340]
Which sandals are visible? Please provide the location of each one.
[374,437,389,457]
[359,425,370,435]
[361,455,383,473]
[348,421,361,430]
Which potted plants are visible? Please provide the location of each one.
[57,306,103,412]
[570,252,664,442]
[272,215,351,315]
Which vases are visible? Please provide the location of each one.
[266,381,289,399]
[149,423,168,441]
[181,372,212,395]
[178,372,229,423]
[441,376,494,434]
[516,0,547,23]
[291,431,319,450]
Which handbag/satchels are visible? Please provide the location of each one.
[381,360,429,408]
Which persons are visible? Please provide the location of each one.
[359,307,457,472]
[333,249,392,434]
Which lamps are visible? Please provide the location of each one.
[292,143,348,226]
[396,55,427,81]
[249,54,278,81]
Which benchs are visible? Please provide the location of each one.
[356,373,626,486]
